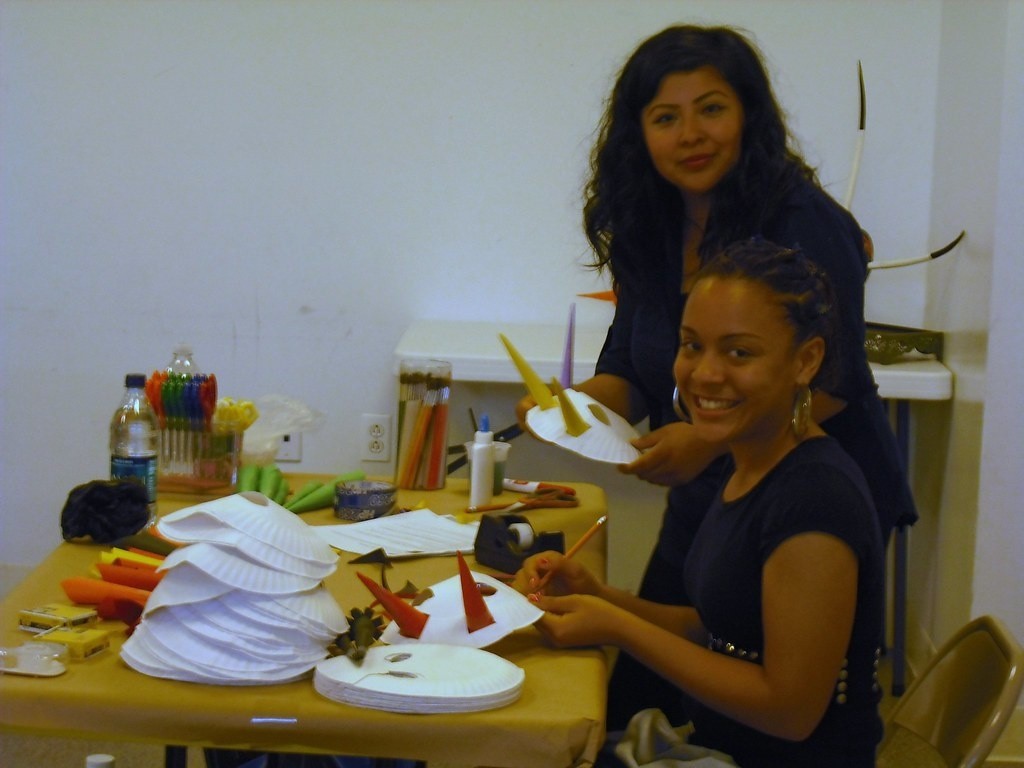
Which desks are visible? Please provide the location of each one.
[391,317,951,695]
[0,471,607,768]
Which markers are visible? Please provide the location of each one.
[503,477,577,496]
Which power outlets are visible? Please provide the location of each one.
[361,412,390,462]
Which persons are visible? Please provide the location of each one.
[517,26,917,724]
[511,240,883,767]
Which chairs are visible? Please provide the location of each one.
[877,616,1023,767]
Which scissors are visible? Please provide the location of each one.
[504,488,579,512]
[145,369,260,488]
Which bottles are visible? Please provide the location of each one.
[470,414,494,509]
[110,373,164,528]
[166,344,199,374]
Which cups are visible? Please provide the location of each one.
[393,355,452,491]
[464,439,511,495]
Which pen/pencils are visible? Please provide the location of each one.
[465,502,515,513]
[533,516,609,596]
[393,371,450,489]
